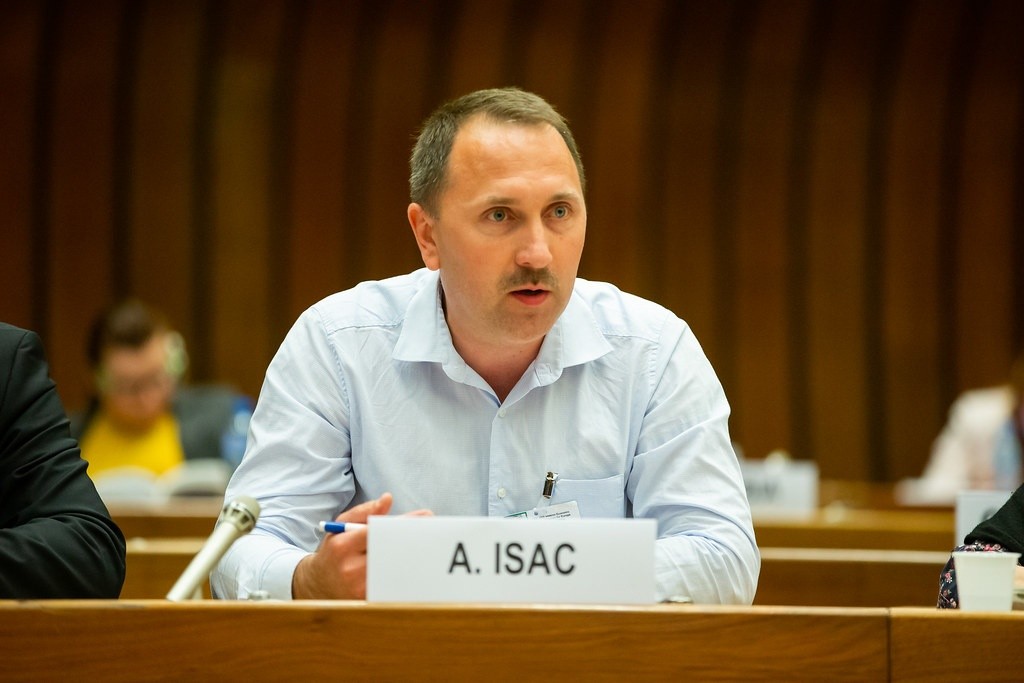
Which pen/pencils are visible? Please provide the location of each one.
[543,470,555,497]
[318,520,367,534]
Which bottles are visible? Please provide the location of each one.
[220,396,253,478]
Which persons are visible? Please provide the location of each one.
[0,318,128,602]
[914,387,1023,508]
[67,297,254,507]
[938,480,1024,614]
[209,89,760,608]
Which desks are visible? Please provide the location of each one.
[119,537,953,607]
[888,607,1024,683]
[0,599,890,683]
[107,498,952,550]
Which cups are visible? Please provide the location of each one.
[952,551,1021,612]
